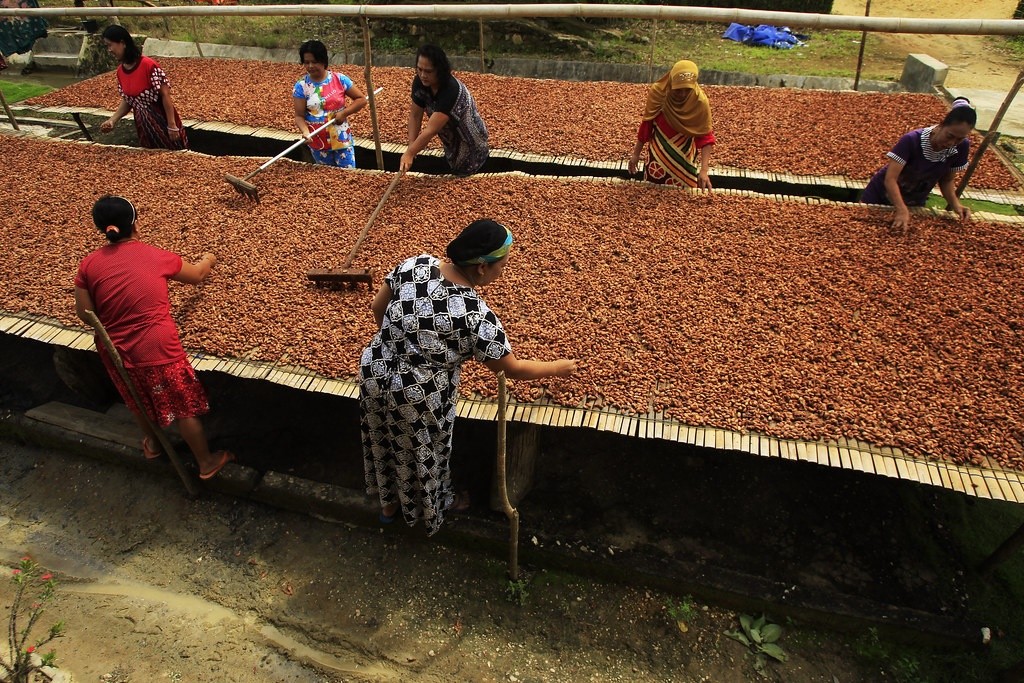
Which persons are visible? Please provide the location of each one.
[75,195,234,478]
[400,46,488,176]
[99,25,189,149]
[861,96,977,234]
[292,40,366,168]
[628,59,715,194]
[358,219,577,538]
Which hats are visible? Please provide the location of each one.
[671,60,699,90]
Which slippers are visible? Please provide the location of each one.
[199,449,237,479]
[450,491,469,512]
[380,505,400,523]
[142,435,161,459]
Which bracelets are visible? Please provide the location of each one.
[167,126,179,132]
